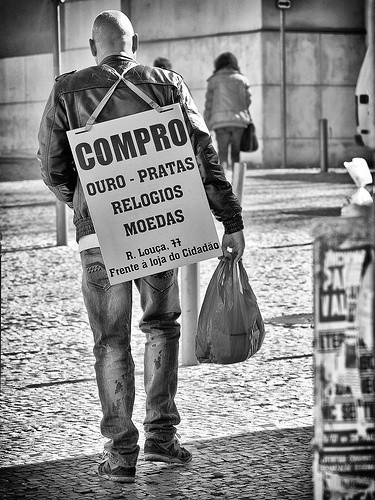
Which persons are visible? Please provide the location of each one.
[34,9,244,482]
[150,56,173,70]
[202,51,251,163]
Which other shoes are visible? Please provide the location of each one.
[97,458,136,482]
[143,433,192,464]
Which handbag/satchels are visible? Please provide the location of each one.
[239,110,258,153]
